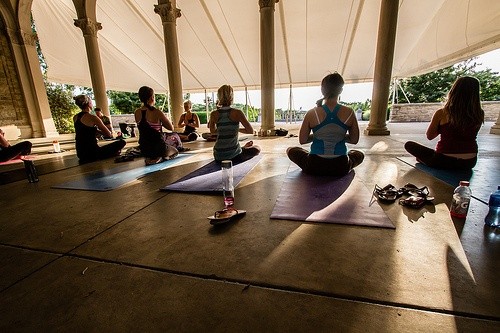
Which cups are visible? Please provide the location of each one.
[267,130,271,136]
[254,129,258,136]
[223,187,235,207]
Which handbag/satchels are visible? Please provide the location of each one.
[274,127,289,136]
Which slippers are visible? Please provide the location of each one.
[208,208,246,226]
[400,182,435,208]
[373,183,403,202]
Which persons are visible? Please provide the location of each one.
[0,129,32,161]
[209,85,260,164]
[95,108,113,132]
[202,102,222,141]
[178,100,200,141]
[135,86,183,154]
[404,76,484,173]
[286,73,364,178]
[73,94,125,160]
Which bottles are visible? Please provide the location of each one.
[221,160,235,188]
[53,140,60,153]
[449,181,473,219]
[484,185,500,226]
[261,129,265,136]
